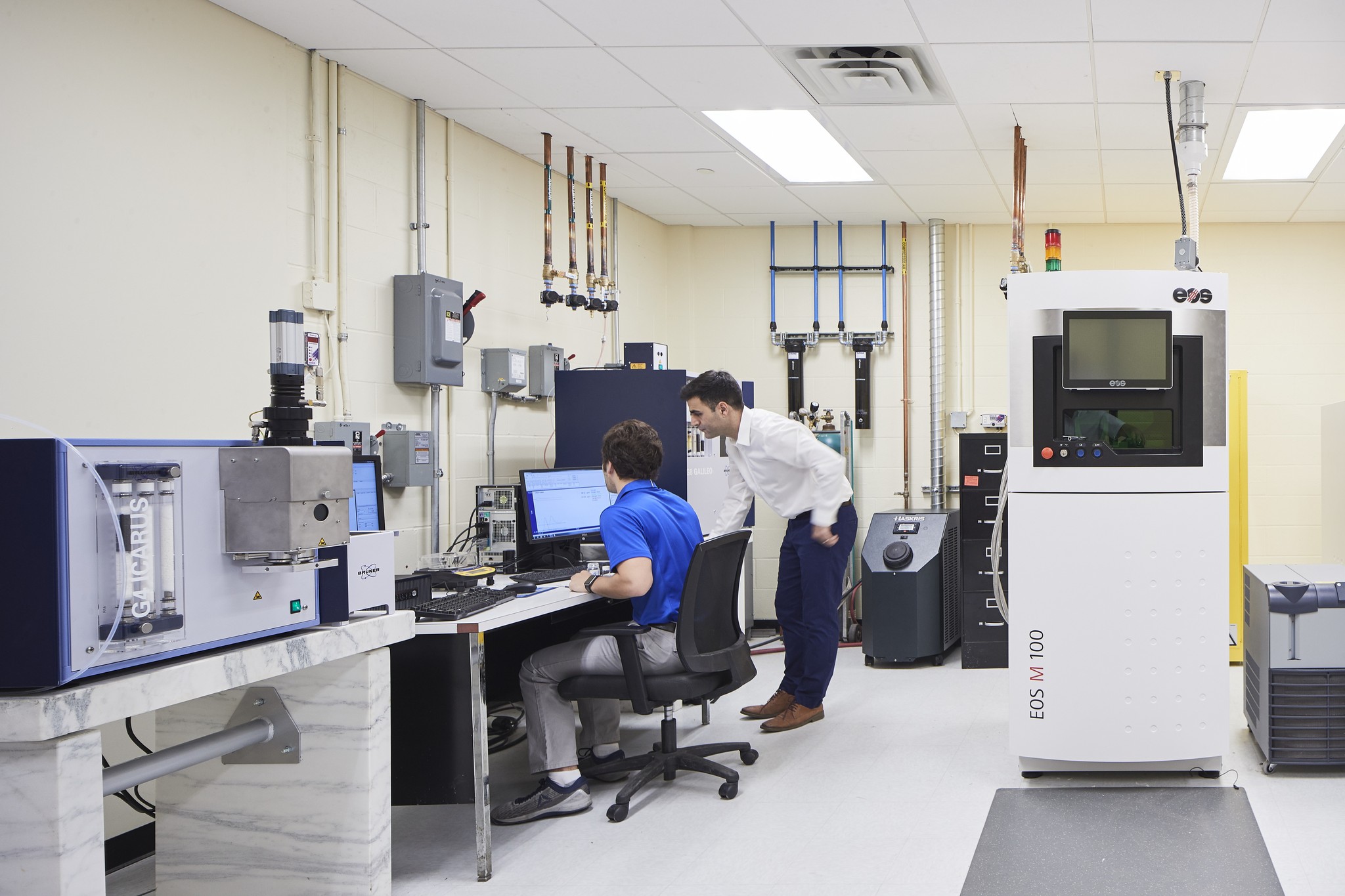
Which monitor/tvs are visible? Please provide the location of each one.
[519,467,619,573]
[1062,310,1173,390]
[349,455,386,531]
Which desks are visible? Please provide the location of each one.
[410,564,622,885]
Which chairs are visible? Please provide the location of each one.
[556,525,761,825]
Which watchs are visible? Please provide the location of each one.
[583,574,601,594]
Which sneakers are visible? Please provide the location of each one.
[490,776,593,826]
[576,746,630,782]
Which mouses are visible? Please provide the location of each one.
[502,582,537,594]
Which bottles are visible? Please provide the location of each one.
[602,566,611,577]
[587,563,600,575]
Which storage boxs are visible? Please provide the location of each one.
[956,429,1010,673]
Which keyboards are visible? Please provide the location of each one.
[509,564,609,585]
[409,586,516,620]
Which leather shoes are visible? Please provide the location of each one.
[740,689,795,718]
[760,696,825,731]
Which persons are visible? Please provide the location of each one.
[678,370,858,732]
[487,419,706,824]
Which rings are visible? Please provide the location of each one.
[823,538,830,544]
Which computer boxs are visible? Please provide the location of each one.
[475,482,582,574]
